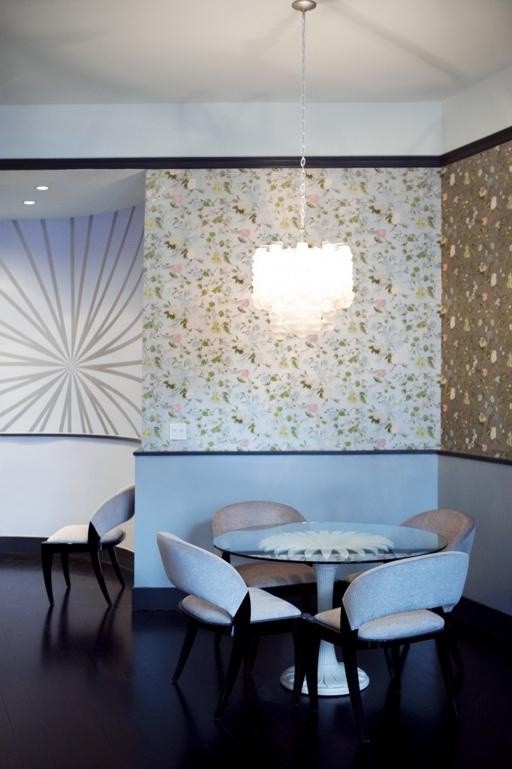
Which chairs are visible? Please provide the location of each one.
[41,484,135,608]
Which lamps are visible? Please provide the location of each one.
[252,0,356,335]
